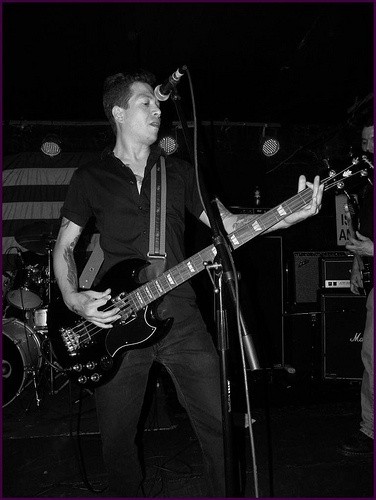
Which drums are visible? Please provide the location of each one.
[6,268,45,311]
[2,317,42,407]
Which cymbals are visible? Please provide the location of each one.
[15,222,83,256]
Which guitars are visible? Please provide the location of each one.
[47,150,375,388]
[344,190,373,297]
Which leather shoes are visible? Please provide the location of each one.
[338,429,376,456]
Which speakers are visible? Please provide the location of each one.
[321,294,368,381]
[189,236,284,369]
[294,251,352,302]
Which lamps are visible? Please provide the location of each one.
[257,125,281,156]
[40,124,63,157]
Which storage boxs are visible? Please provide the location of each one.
[317,288,367,381]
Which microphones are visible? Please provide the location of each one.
[17,249,26,268]
[154,65,187,102]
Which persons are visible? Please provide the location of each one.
[359,284,375,445]
[344,122,375,296]
[52,72,326,500]
